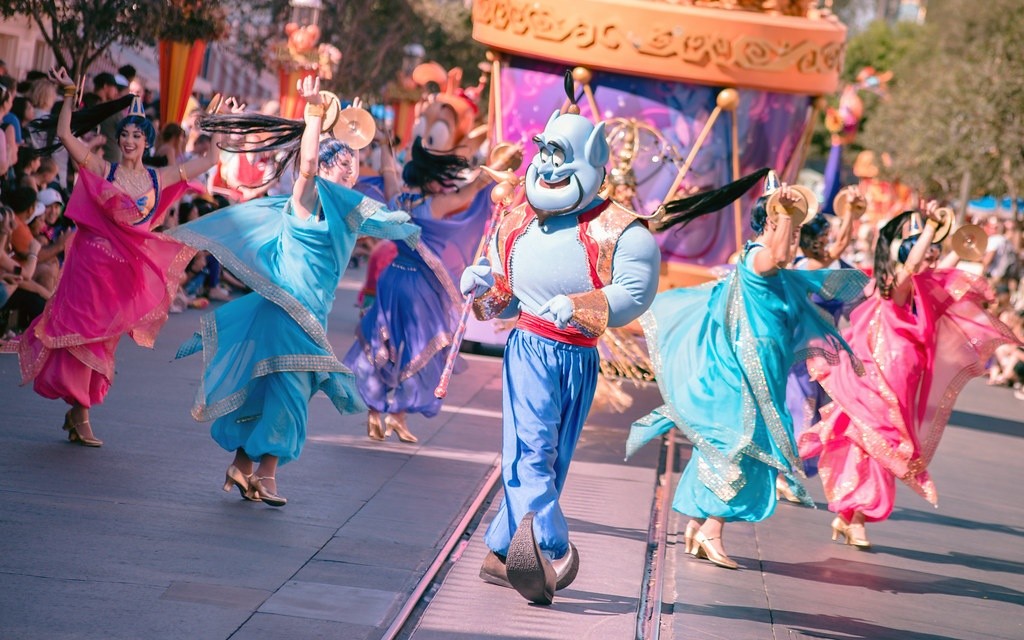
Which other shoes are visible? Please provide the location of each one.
[479,541,580,591]
[173,277,245,313]
[506,511,558,605]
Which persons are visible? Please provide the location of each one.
[831,216,1024,399]
[165,77,421,506]
[18,65,225,447]
[344,132,529,442]
[0,60,410,354]
[774,188,864,506]
[622,182,871,569]
[799,203,1023,546]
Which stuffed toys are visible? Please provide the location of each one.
[356,68,486,310]
[461,105,666,604]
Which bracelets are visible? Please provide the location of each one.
[64,86,77,96]
[307,103,323,117]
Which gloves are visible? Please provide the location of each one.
[459,254,494,299]
[535,292,574,329]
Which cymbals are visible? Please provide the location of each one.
[928,204,955,243]
[952,224,988,260]
[201,93,226,123]
[72,73,86,106]
[768,183,820,227]
[832,187,868,220]
[303,90,377,150]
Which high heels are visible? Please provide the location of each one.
[830,516,850,541]
[774,474,803,503]
[367,410,385,441]
[684,523,709,560]
[63,411,103,447]
[244,473,288,507]
[222,465,262,502]
[845,523,872,547]
[382,415,418,443]
[689,528,739,568]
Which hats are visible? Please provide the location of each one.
[36,188,67,211]
[24,204,46,222]
